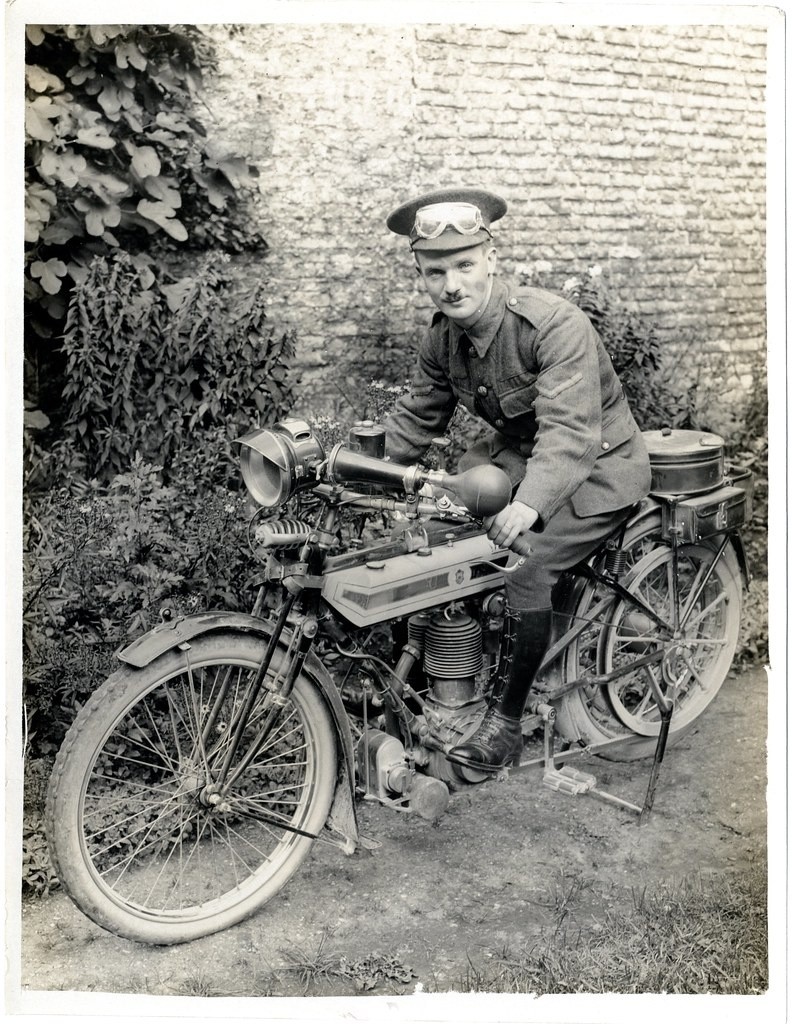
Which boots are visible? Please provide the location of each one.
[337,615,428,719]
[445,607,553,771]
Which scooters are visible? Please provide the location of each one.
[43,414,755,950]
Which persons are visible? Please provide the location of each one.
[360,189,650,771]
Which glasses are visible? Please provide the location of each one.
[409,200,485,240]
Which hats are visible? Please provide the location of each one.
[386,188,507,250]
